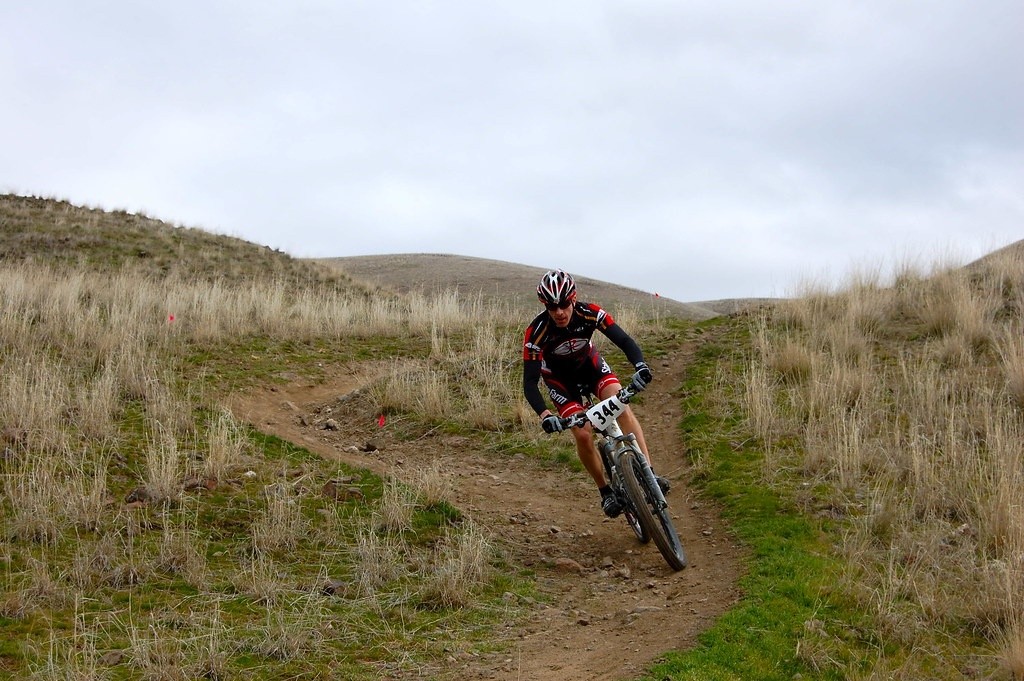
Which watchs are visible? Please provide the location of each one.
[635,362,650,369]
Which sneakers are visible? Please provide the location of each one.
[654,474,670,494]
[601,492,620,518]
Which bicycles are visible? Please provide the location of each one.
[557,381,686,570]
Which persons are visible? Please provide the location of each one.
[523,277,670,518]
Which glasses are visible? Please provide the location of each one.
[545,300,571,310]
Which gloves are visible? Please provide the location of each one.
[631,360,652,391]
[541,414,563,433]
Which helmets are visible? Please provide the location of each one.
[537,269,576,304]
